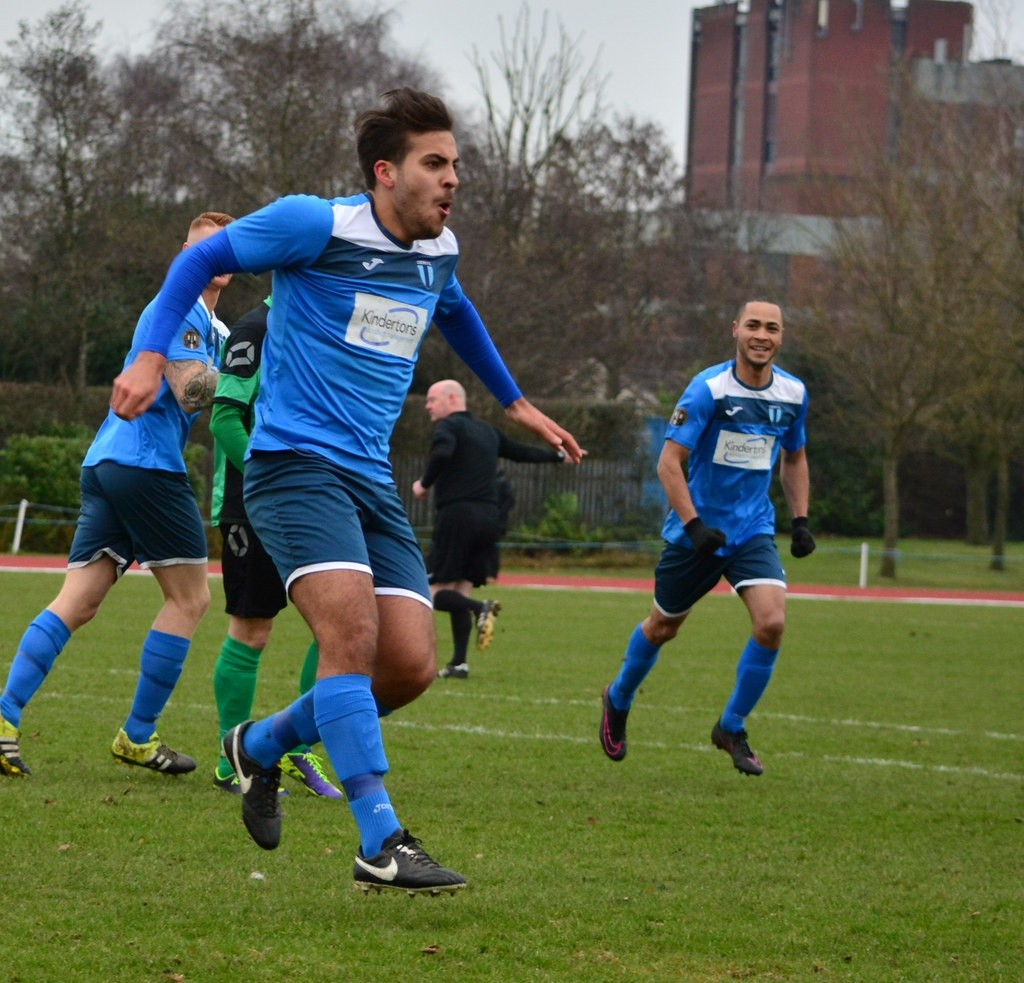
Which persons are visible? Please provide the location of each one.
[410,378,590,679]
[207,294,344,803]
[597,289,817,777]
[107,90,590,897]
[1,211,238,776]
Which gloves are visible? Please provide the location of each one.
[790,516,815,558]
[683,517,726,557]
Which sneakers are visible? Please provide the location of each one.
[711,715,762,776]
[475,599,502,651]
[276,747,344,799]
[109,727,197,774]
[436,662,469,678]
[353,827,468,899]
[221,720,282,850]
[599,684,631,761]
[212,766,290,797]
[0,713,32,778]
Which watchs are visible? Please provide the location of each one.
[554,448,566,466]
